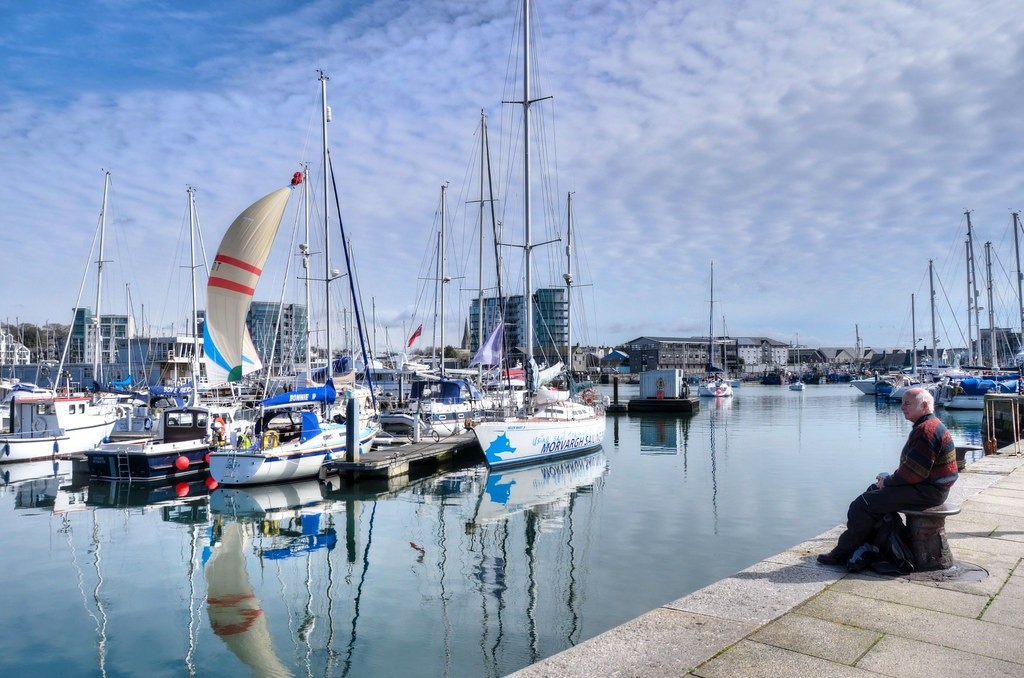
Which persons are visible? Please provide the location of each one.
[817,387,959,565]
[237,429,252,449]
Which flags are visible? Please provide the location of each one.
[471,322,502,366]
[408,325,422,347]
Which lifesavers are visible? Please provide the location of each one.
[582,389,595,402]
[144,418,153,430]
[955,386,963,393]
[214,417,226,435]
[656,379,664,389]
[116,407,124,417]
[263,429,279,448]
[32,418,46,434]
[154,407,163,417]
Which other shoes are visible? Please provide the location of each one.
[817,545,850,565]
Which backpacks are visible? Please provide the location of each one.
[874,512,917,575]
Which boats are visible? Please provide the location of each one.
[759,331,860,386]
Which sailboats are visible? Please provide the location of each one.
[469,0,609,470]
[721,315,741,387]
[1,68,570,487]
[698,258,734,397]
[788,332,805,390]
[848,210,1024,411]
